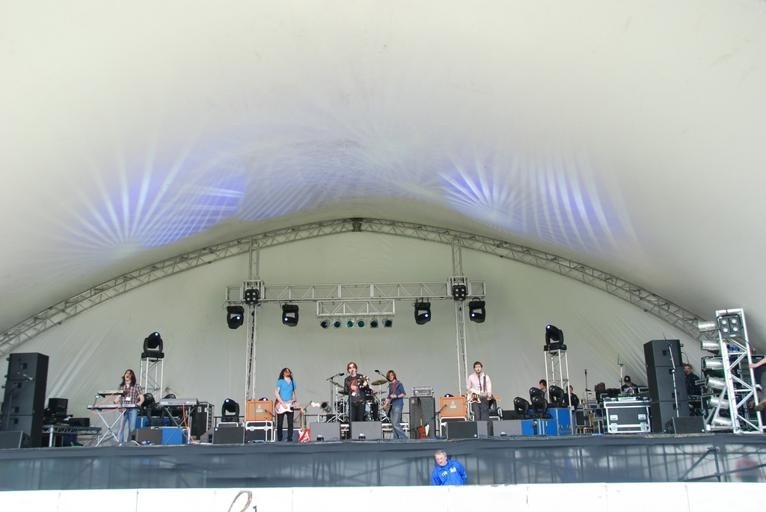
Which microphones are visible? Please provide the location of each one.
[338,371,345,377]
[375,369,381,374]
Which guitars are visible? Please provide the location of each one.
[467,389,503,404]
[417,397,430,439]
[383,380,401,410]
[274,399,312,414]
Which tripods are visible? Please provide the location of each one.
[377,382,390,423]
[326,374,346,424]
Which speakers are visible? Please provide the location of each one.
[211,426,245,446]
[718,314,741,338]
[135,428,163,446]
[409,396,436,439]
[310,422,342,442]
[643,339,706,435]
[0,352,49,449]
[446,421,478,439]
[351,421,384,440]
[190,402,212,441]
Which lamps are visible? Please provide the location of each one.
[543,323,567,351]
[141,329,165,362]
[220,397,240,424]
[226,289,300,329]
[697,307,764,437]
[413,283,486,326]
[512,384,566,418]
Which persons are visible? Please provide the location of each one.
[746,384,765,417]
[429,449,469,487]
[271,367,298,443]
[112,369,144,447]
[465,360,492,422]
[383,369,408,440]
[748,357,766,372]
[619,374,639,394]
[538,378,551,405]
[563,385,580,410]
[342,361,369,422]
[682,364,707,395]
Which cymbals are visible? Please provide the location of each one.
[373,379,385,386]
[338,390,347,394]
[375,391,386,394]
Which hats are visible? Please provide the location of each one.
[624,376,630,383]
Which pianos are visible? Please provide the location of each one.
[160,398,199,406]
[87,390,139,410]
